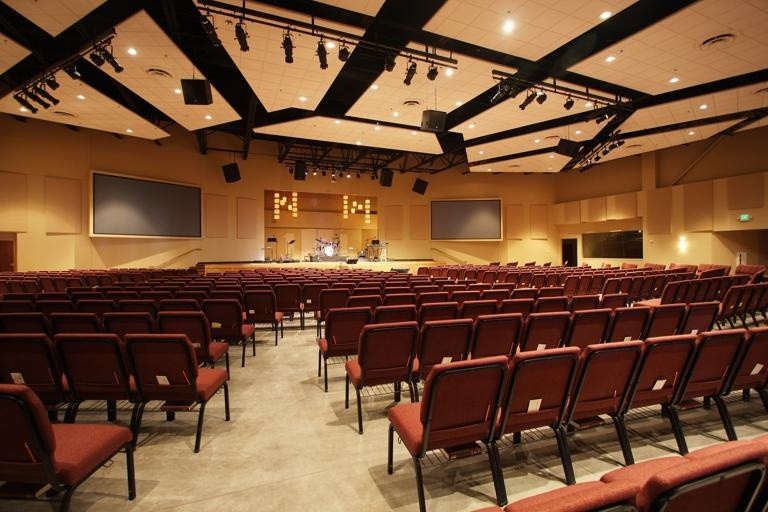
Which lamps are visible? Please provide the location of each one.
[284,162,377,183]
[491,66,636,124]
[577,127,624,173]
[194,0,459,85]
[11,24,126,115]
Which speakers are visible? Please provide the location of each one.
[379,169,394,186]
[221,162,241,183]
[555,138,580,157]
[294,160,306,180]
[181,79,213,106]
[421,110,447,132]
[412,178,429,194]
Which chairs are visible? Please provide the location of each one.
[2,334,71,422]
[495,347,581,502]
[204,299,255,366]
[724,328,768,424]
[570,308,613,344]
[387,355,507,512]
[274,284,302,330]
[472,314,521,359]
[317,307,373,394]
[626,335,697,457]
[678,329,744,446]
[158,311,230,384]
[565,340,644,470]
[315,289,349,339]
[345,321,420,433]
[521,310,570,354]
[127,333,230,453]
[351,261,768,331]
[2,384,137,511]
[245,290,283,346]
[215,267,357,298]
[613,306,649,341]
[301,283,329,330]
[413,318,474,402]
[4,269,215,333]
[56,334,140,430]
